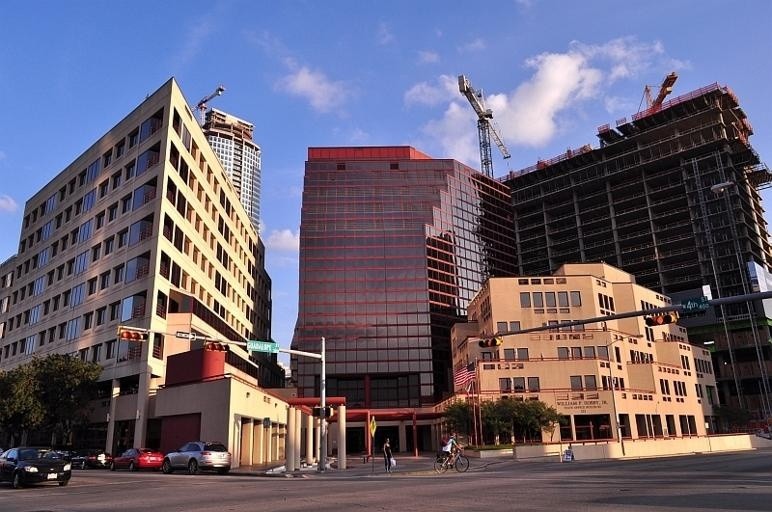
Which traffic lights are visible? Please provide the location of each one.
[645,312,678,327]
[478,336,504,348]
[120,329,148,343]
[203,342,230,353]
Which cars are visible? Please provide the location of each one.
[0,447,71,489]
[62,447,164,473]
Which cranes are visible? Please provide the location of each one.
[458,74,512,178]
[190,83,226,127]
[645,72,679,109]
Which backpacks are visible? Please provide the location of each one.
[440,437,453,446]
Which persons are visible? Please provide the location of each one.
[440,431,464,469]
[382,435,394,473]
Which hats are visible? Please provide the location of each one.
[450,432,457,437]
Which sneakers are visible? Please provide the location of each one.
[385,469,392,473]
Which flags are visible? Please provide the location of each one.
[452,360,477,387]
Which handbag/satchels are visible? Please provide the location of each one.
[390,458,396,467]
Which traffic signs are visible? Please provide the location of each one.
[247,340,279,353]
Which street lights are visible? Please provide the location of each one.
[605,335,644,456]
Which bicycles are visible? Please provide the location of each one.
[433,447,470,473]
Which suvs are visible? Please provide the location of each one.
[162,442,232,475]
